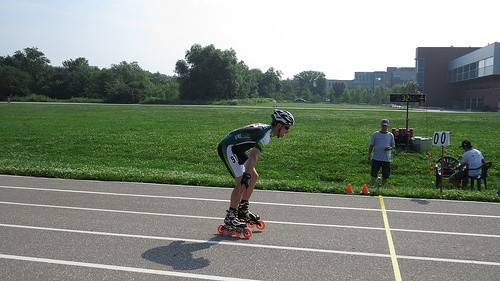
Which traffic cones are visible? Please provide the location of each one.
[360,184,370,195]
[345,184,354,195]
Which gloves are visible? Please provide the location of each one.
[241,172,251,189]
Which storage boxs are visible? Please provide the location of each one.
[411,136,433,152]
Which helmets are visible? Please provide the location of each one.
[271,109,295,127]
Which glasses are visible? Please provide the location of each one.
[284,126,290,130]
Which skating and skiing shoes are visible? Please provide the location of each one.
[218,210,252,238]
[237,201,265,230]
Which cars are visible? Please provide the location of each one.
[294,98,306,103]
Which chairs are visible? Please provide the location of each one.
[435,156,460,187]
[459,161,492,192]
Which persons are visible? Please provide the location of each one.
[449,139,486,190]
[368,119,395,186]
[217,110,295,238]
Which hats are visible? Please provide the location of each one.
[459,140,473,147]
[381,119,389,126]
